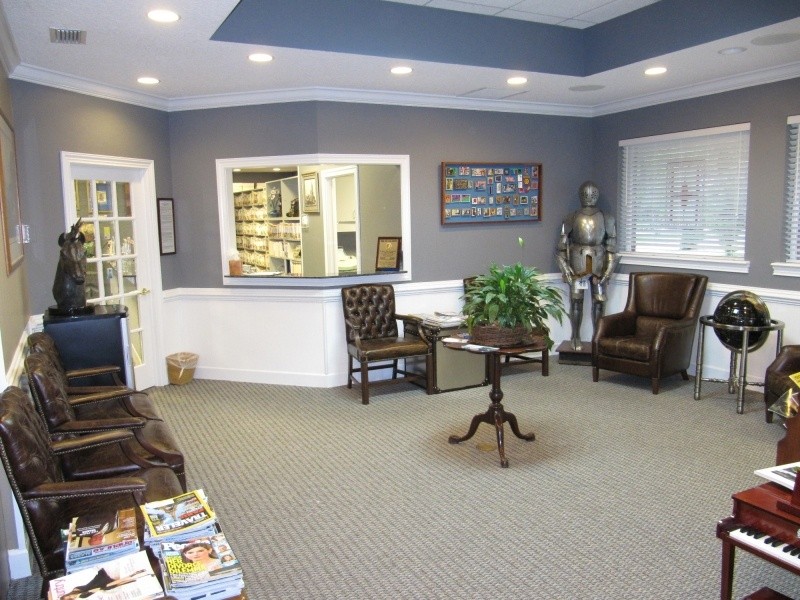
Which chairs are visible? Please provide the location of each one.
[341,284,434,404]
[0,331,185,600]
[591,272,709,396]
[764,344,800,425]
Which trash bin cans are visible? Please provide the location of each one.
[168,353,198,385]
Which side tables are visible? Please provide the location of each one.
[42,304,136,391]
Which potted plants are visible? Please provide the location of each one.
[455,237,566,351]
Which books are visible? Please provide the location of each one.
[47,489,245,600]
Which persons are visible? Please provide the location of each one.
[220,555,238,567]
[555,181,616,350]
[75,511,119,547]
[59,568,146,600]
[217,545,231,555]
[180,543,221,571]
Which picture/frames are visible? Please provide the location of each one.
[301,172,319,213]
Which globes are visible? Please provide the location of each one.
[712,290,770,353]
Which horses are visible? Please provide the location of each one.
[51,231,88,308]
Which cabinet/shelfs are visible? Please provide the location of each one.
[233,177,303,277]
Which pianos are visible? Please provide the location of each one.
[716,405,800,600]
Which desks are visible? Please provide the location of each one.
[405,312,487,393]
[440,332,554,468]
